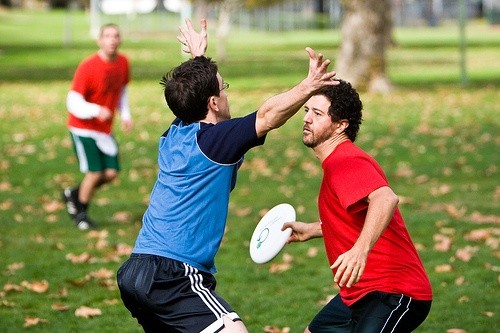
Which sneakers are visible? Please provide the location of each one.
[64,189,79,217]
[73,211,90,231]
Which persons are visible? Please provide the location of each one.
[62,22,134,231]
[117,17,341,333]
[303,78,433,333]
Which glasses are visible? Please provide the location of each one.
[208,83,229,103]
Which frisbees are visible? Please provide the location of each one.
[250,203,296,265]
